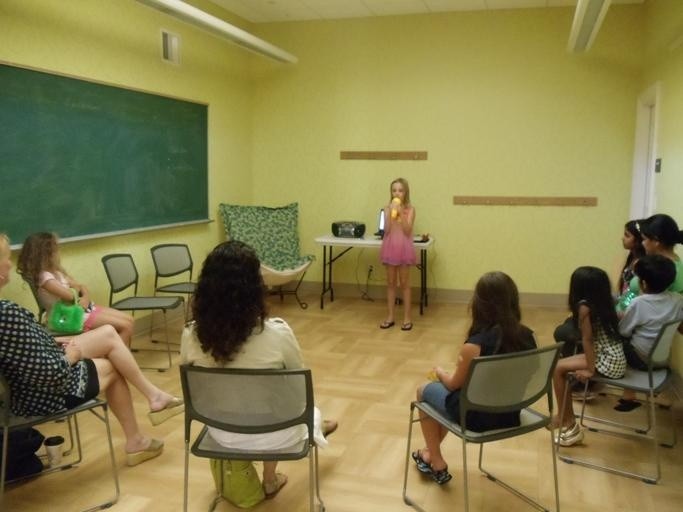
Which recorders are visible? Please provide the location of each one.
[332,221,366,238]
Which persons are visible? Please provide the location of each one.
[0,233,185,467]
[411,271,538,484]
[16,232,136,343]
[543,214,682,430]
[181,240,339,498]
[379,179,416,330]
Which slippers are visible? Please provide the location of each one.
[401,322,412,330]
[411,450,451,485]
[380,321,394,328]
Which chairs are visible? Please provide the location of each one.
[25,275,77,338]
[0,368,120,512]
[554,319,682,484]
[99,251,187,371]
[402,339,564,511]
[146,242,199,348]
[177,362,325,511]
[218,199,316,312]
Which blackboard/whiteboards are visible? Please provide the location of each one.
[0,60,216,252]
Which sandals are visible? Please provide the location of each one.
[261,474,286,496]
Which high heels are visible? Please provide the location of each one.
[147,397,184,425]
[554,424,583,446]
[126,439,162,467]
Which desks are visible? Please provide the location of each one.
[314,231,433,315]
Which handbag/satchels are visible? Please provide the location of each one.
[46,300,84,334]
[209,458,265,509]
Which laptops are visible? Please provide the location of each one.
[375,209,384,235]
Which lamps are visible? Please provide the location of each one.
[142,1,300,66]
[565,1,615,54]
